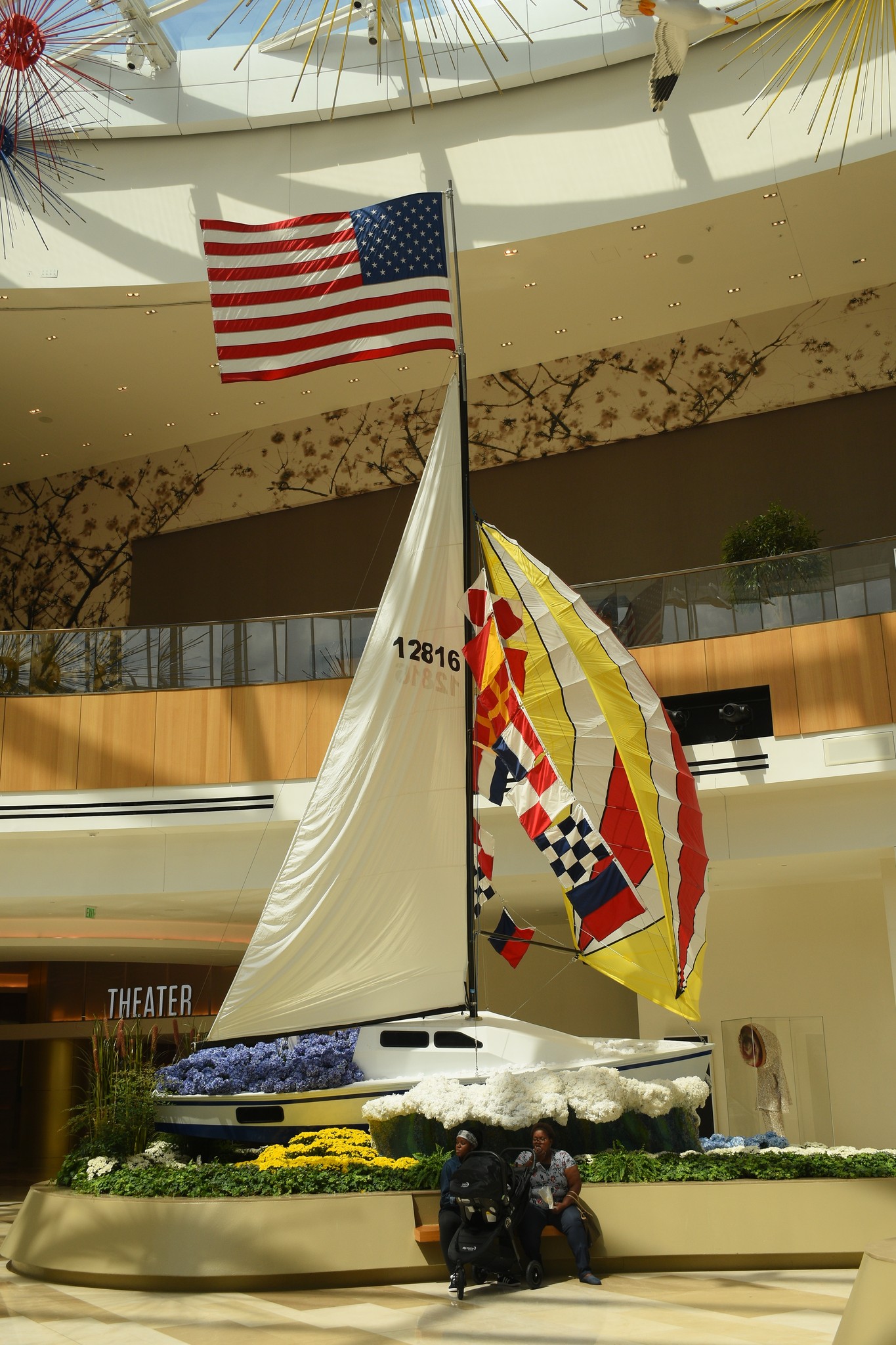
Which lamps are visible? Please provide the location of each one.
[366,7,389,45]
[665,709,687,731]
[352,0,367,10]
[86,0,104,12]
[719,703,753,724]
[126,33,145,71]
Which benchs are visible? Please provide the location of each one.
[414,1224,566,1242]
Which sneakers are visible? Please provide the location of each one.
[497,1273,521,1286]
[449,1272,466,1292]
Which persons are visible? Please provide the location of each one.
[438,1125,521,1291]
[513,1123,602,1285]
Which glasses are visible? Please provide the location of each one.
[532,1135,550,1143]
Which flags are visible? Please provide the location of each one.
[199,190,456,383]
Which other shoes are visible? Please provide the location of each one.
[580,1275,601,1285]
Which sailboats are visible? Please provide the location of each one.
[128,182,728,1155]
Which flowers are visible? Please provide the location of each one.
[56,1010,896,1198]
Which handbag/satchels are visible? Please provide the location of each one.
[566,1191,600,1247]
